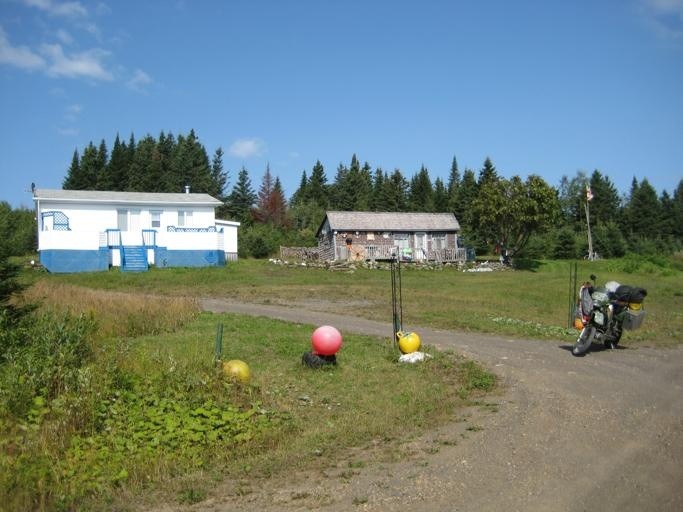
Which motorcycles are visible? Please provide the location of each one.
[571,274,648,356]
